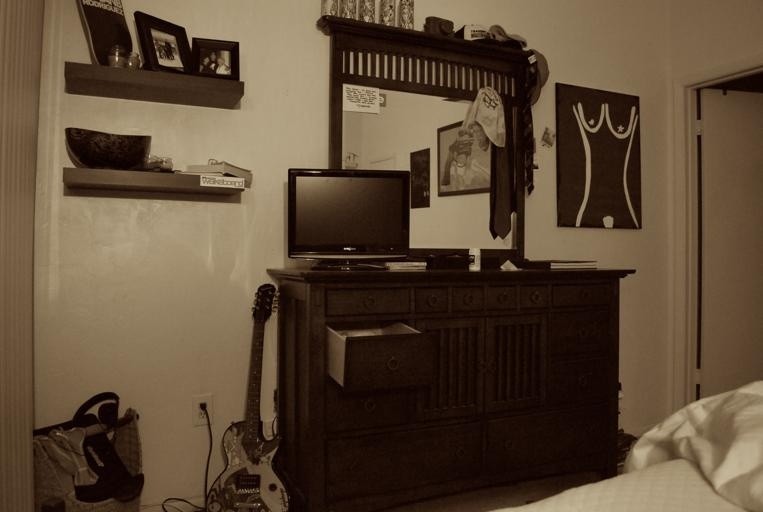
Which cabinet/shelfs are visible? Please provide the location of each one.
[60,60,246,195]
[265,264,636,511]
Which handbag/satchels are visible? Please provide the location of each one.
[33,408,142,512]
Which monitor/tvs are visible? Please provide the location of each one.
[287,168,411,268]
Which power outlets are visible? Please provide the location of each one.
[191,393,212,426]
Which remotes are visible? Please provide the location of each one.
[385,261,428,267]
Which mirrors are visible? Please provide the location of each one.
[317,16,528,262]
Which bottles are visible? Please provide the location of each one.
[108,45,139,69]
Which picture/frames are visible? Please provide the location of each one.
[435,120,491,197]
[132,10,240,81]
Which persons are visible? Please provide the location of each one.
[207,51,231,74]
[198,56,209,73]
[215,57,230,74]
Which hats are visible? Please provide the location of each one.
[528,48,549,104]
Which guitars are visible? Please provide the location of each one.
[207,284,292,512]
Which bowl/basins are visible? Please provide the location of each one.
[63,126,153,172]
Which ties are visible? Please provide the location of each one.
[494,147,511,238]
[490,146,499,239]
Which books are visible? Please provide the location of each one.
[184,160,252,183]
[173,169,250,188]
[524,265,598,271]
[525,258,598,265]
